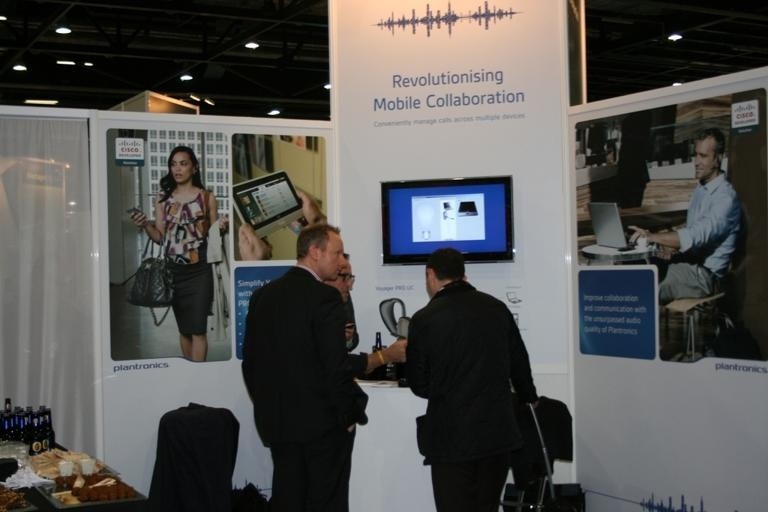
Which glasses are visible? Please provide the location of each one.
[338,273,356,284]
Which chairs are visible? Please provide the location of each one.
[147,403,241,506]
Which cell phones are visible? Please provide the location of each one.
[126,207,144,216]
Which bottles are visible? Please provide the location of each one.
[372,331,386,380]
[0,398,55,455]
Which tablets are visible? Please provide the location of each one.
[232,169,305,239]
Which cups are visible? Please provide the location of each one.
[638,237,649,247]
[59,461,73,476]
[81,459,94,476]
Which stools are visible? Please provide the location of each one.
[666,292,727,361]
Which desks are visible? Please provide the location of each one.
[575,180,697,259]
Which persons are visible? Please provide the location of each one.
[238,189,327,261]
[241,224,409,512]
[627,127,742,301]
[129,146,229,362]
[407,248,539,512]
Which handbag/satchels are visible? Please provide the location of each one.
[127,228,176,307]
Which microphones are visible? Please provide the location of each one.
[396,317,412,378]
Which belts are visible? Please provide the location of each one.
[700,261,725,281]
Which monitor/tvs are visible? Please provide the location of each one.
[380,174,515,266]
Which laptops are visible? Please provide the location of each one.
[588,202,659,249]
